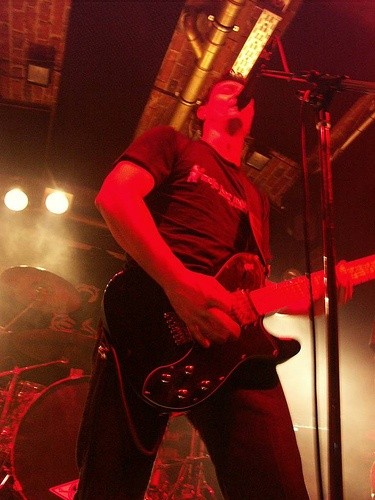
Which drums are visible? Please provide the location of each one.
[0,367,91,500]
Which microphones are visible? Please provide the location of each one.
[237,42,273,107]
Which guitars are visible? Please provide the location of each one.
[102,251,375,412]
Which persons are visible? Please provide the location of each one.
[73,73,355,500]
[0,299,94,382]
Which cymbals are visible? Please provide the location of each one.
[14,329,98,362]
[0,265,82,314]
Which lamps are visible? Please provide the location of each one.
[229,5,283,80]
[26,61,63,88]
[45,190,70,215]
[4,186,28,212]
